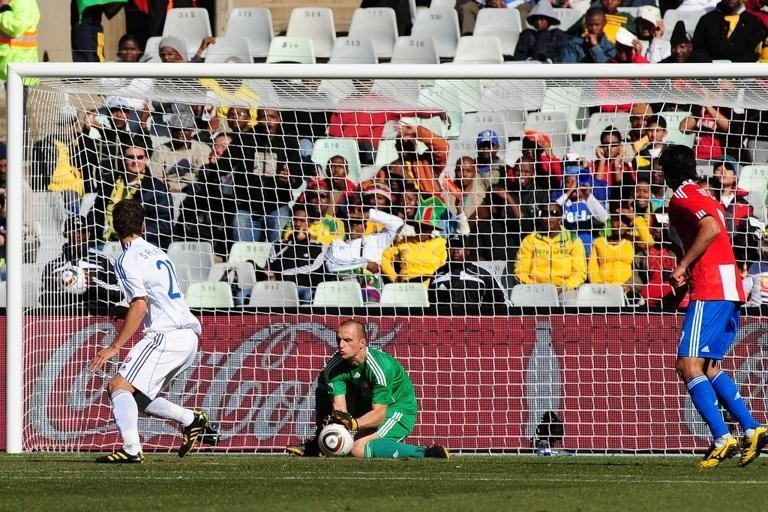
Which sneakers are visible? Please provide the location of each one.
[179,411,208,458]
[288,441,323,457]
[738,427,768,468]
[426,444,449,459]
[696,434,739,469]
[96,449,146,463]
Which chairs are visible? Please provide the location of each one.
[1,5,766,308]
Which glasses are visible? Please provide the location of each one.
[127,156,144,159]
[541,211,560,217]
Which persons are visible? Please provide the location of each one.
[1,2,767,307]
[286,318,450,460]
[660,146,767,469]
[88,200,209,463]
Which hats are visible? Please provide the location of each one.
[446,234,470,247]
[477,130,498,145]
[407,194,447,231]
[671,21,692,43]
[526,0,561,25]
[365,183,391,200]
[632,6,661,27]
[168,112,195,129]
[714,155,738,173]
[106,98,129,107]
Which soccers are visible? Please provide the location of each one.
[318,422,355,455]
[59,265,86,295]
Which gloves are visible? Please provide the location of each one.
[332,411,359,430]
[321,415,332,427]
